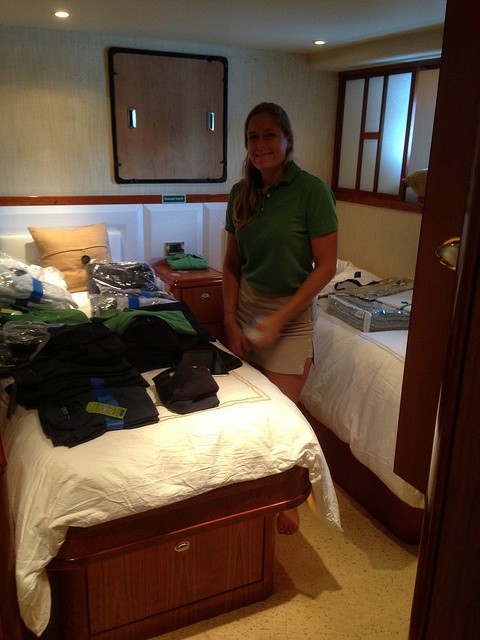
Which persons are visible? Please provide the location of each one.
[221,102,339,535]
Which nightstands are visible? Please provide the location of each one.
[150,263,223,342]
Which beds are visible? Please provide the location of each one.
[1,232,345,638]
[298,257,442,545]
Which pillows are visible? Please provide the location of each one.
[24,224,112,293]
